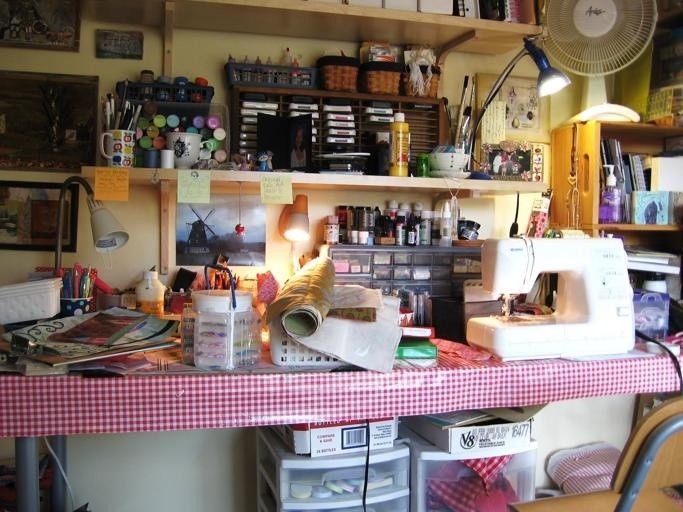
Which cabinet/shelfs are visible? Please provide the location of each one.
[551,122,683,239]
[256,426,537,512]
[79,1,545,191]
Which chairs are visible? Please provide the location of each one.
[508,397,682,511]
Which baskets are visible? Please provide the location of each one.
[317,56,357,91]
[365,61,402,95]
[224,62,316,89]
[404,64,441,98]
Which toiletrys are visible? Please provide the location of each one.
[599,164,623,224]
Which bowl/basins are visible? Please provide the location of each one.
[427,153,470,172]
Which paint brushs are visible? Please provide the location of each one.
[453,75,470,148]
[459,104,472,142]
[442,96,453,128]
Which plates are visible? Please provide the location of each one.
[452,239,484,247]
[428,170,473,178]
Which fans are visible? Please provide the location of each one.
[538,0,658,124]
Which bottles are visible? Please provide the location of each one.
[458,219,481,240]
[136,271,164,320]
[531,149,544,182]
[323,200,452,247]
[416,153,429,176]
[180,287,261,372]
[227,54,272,84]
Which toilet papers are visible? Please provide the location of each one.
[267,257,338,338]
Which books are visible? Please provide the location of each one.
[0,304,183,370]
[47,311,151,350]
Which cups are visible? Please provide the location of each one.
[142,132,214,168]
[99,129,135,167]
[59,297,94,316]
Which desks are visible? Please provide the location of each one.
[0,337,682,511]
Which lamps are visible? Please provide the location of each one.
[278,194,311,263]
[54,175,128,269]
[465,37,572,179]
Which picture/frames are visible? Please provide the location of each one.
[476,71,551,184]
[0,0,101,253]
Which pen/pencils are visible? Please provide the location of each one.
[59,263,97,301]
[101,91,142,131]
